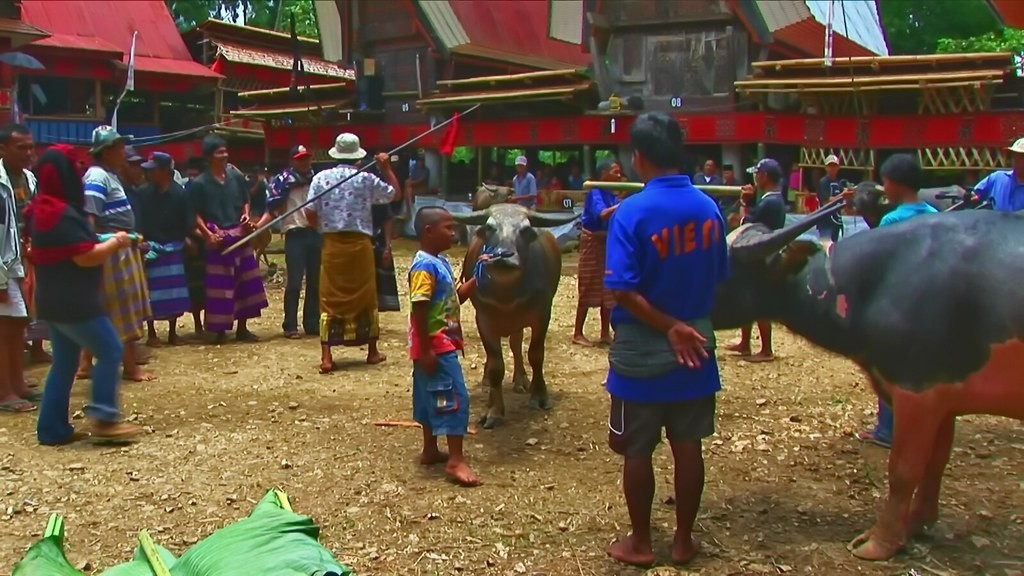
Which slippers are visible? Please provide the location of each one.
[0,389,44,412]
[857,430,893,450]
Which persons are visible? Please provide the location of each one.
[0,111,818,564]
[969,136,1024,212]
[854,154,939,447]
[816,154,851,244]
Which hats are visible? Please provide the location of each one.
[88,125,133,154]
[124,145,143,162]
[141,151,175,172]
[746,158,783,178]
[824,154,840,166]
[514,155,529,167]
[1004,137,1024,153]
[289,144,313,159]
[328,132,367,161]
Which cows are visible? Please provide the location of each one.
[712,196,1023,560]
[448,203,582,430]
[472,182,515,211]
[840,178,967,229]
